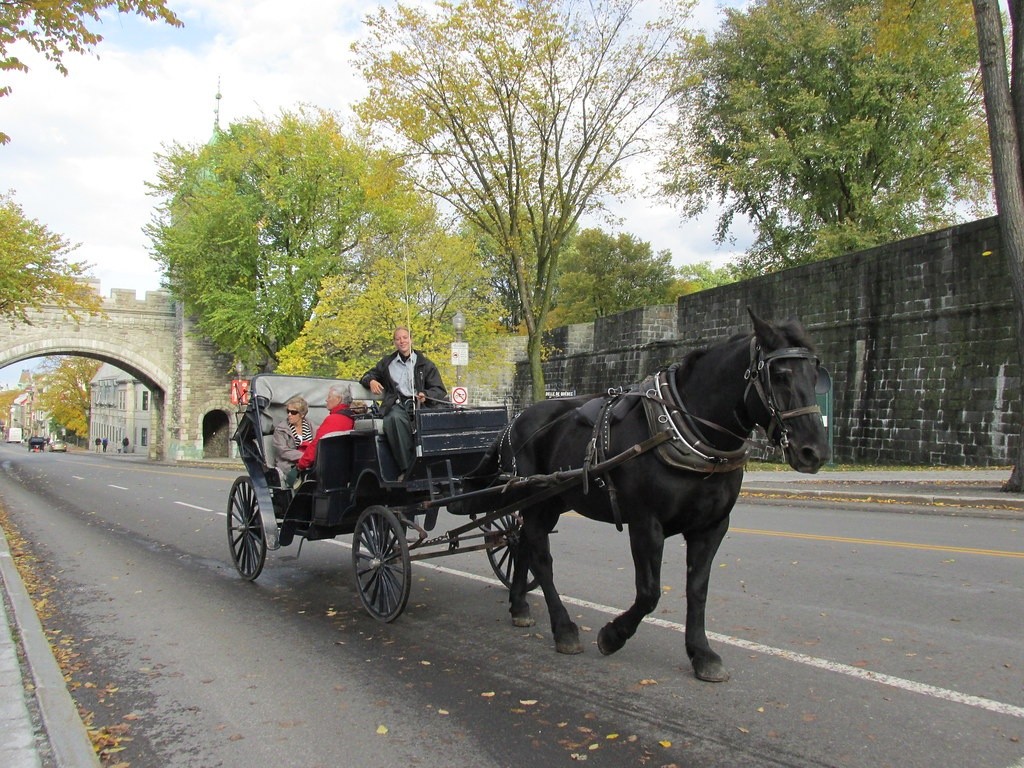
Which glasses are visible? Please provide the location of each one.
[287,409,299,415]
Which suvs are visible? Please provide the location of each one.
[28,437,45,452]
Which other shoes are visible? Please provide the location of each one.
[398,472,406,481]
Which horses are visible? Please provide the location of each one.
[470,302,830,683]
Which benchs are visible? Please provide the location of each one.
[230,374,385,541]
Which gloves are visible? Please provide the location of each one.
[286,465,299,486]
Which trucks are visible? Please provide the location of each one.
[5,427,22,443]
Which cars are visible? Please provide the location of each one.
[48,440,67,452]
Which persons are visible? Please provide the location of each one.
[102,437,108,452]
[359,327,447,481]
[95,438,101,453]
[272,397,316,492]
[25,432,27,443]
[47,435,53,446]
[123,436,129,453]
[116,438,122,453]
[297,384,355,471]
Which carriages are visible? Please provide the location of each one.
[224,309,833,687]
